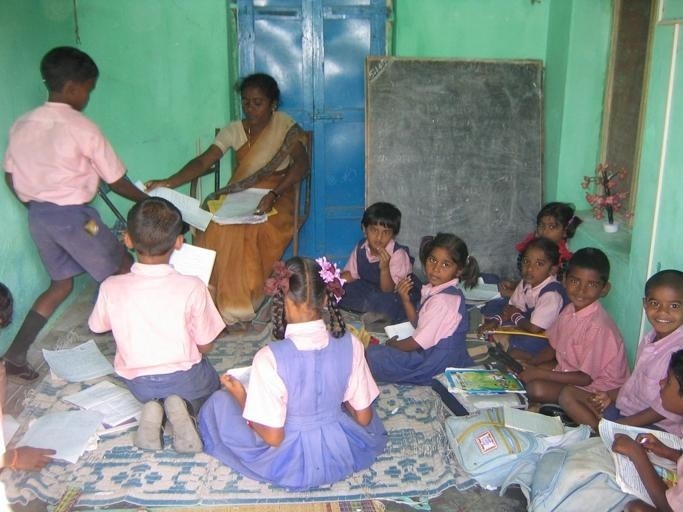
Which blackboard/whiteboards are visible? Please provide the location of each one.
[365,56,545,332]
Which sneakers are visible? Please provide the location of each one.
[0,356,40,385]
[537,403,580,427]
[134,399,166,450]
[163,394,205,454]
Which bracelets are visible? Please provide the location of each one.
[490,315,502,326]
[245,420,255,429]
[511,313,526,326]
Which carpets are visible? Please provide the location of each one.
[0,322,477,505]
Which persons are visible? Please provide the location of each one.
[613,349,683,512]
[335,202,423,324]
[558,270,683,439]
[366,233,480,387]
[87,197,227,454]
[515,247,631,431]
[0,282,56,512]
[480,202,582,327]
[478,237,572,365]
[197,257,388,491]
[2,47,151,380]
[144,74,310,334]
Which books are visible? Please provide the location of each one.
[1,338,141,465]
[444,366,564,437]
[385,321,416,341]
[458,276,501,307]
[597,417,683,509]
[134,179,275,287]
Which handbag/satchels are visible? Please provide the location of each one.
[498,436,638,511]
[445,407,541,490]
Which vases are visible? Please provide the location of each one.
[604,223,618,233]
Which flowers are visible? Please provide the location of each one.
[265,262,292,295]
[315,256,346,302]
[559,240,572,266]
[581,163,633,223]
[517,233,536,252]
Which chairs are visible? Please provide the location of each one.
[189,130,314,257]
